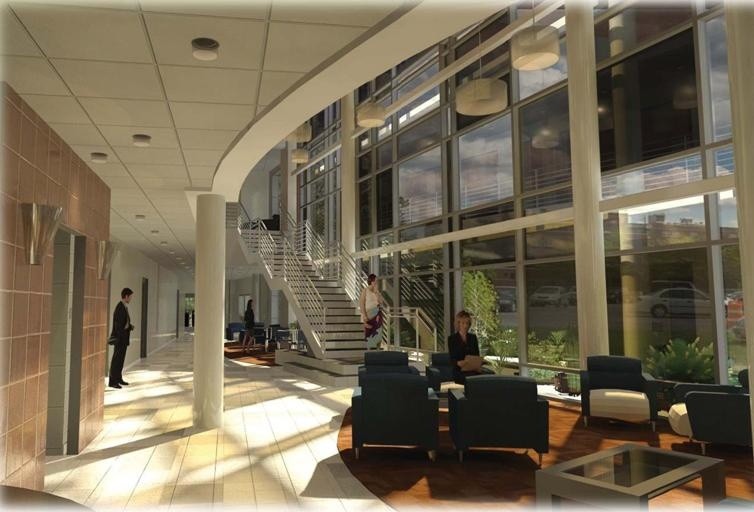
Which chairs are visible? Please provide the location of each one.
[667,369,752,455]
[580,356,658,433]
[268,324,292,341]
[351,349,549,467]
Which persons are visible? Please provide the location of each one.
[448,310,482,385]
[359,274,384,351]
[243,299,256,346]
[108,287,134,389]
[192,309,195,327]
[185,311,189,327]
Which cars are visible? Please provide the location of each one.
[492,286,577,312]
[724,289,743,318]
[639,281,728,318]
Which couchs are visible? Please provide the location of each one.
[226,322,264,340]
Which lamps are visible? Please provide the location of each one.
[91,153,107,164]
[291,127,308,165]
[356,80,385,129]
[132,134,150,147]
[511,0,560,72]
[598,72,613,132]
[192,38,220,61]
[21,202,63,265]
[531,97,560,150]
[95,239,120,281]
[671,44,697,110]
[453,22,508,117]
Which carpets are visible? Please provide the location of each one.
[336,394,754,511]
[224,341,282,366]
